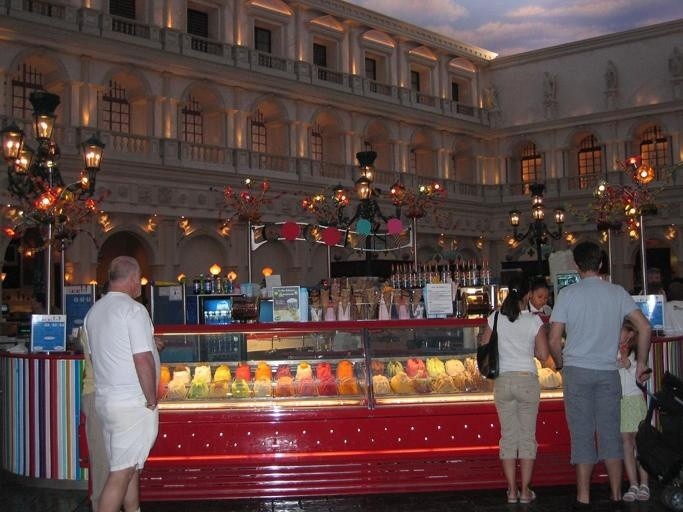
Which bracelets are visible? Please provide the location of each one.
[556,364,563,371]
[147,403,157,406]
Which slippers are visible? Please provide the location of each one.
[506,488,535,504]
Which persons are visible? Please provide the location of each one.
[550,242,651,512]
[525,277,553,317]
[479,276,549,503]
[84,256,161,512]
[617,317,649,502]
[80,280,169,512]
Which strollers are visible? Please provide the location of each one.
[634,368,683,512]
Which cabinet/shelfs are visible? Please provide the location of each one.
[78,316,642,502]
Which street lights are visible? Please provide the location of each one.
[593,155,677,297]
[507,183,565,274]
[0,88,106,323]
[332,150,404,261]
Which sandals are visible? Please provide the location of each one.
[637,484,650,500]
[623,485,637,502]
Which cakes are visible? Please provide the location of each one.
[158,358,493,400]
[533,357,562,388]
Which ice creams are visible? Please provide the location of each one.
[311,277,423,315]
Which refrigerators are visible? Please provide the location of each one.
[196,294,245,362]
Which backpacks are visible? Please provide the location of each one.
[636,421,682,486]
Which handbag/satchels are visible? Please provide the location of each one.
[477,328,499,378]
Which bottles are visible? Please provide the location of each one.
[203,311,239,360]
[453,288,464,319]
[389,256,492,288]
[192,271,235,294]
